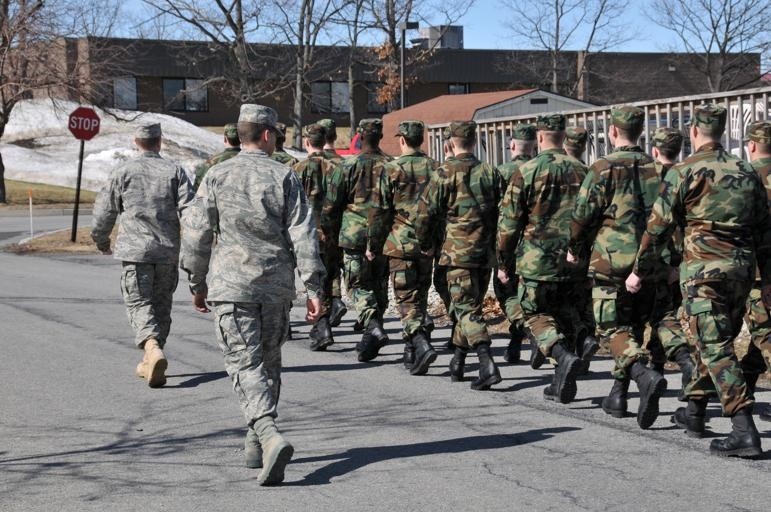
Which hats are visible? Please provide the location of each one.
[649,127,683,147]
[441,127,451,141]
[357,118,384,135]
[134,120,162,139]
[301,123,329,141]
[317,119,337,136]
[534,113,566,132]
[450,121,477,138]
[223,122,239,138]
[237,103,287,138]
[510,124,536,140]
[564,127,588,147]
[609,106,645,131]
[684,104,727,130]
[393,120,424,138]
[742,119,771,145]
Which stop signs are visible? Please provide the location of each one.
[69,106,101,139]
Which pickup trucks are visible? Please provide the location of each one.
[335,133,362,156]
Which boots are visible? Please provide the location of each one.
[709,407,763,458]
[309,315,334,351]
[253,413,296,487]
[601,378,630,418]
[650,362,665,376]
[422,313,434,342]
[243,425,264,469]
[673,397,709,438]
[449,347,468,382]
[522,329,545,369]
[470,342,501,391]
[356,314,390,362]
[136,338,169,388]
[443,321,457,349]
[549,340,582,404]
[576,330,600,376]
[402,337,416,368]
[328,296,347,327]
[739,355,759,392]
[354,316,366,333]
[627,360,668,430]
[408,329,438,375]
[544,365,560,402]
[672,347,698,401]
[502,330,525,362]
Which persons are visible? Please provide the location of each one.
[181,103,329,486]
[91,120,196,389]
[267,121,300,339]
[192,121,240,201]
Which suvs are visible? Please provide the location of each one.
[583,115,747,161]
